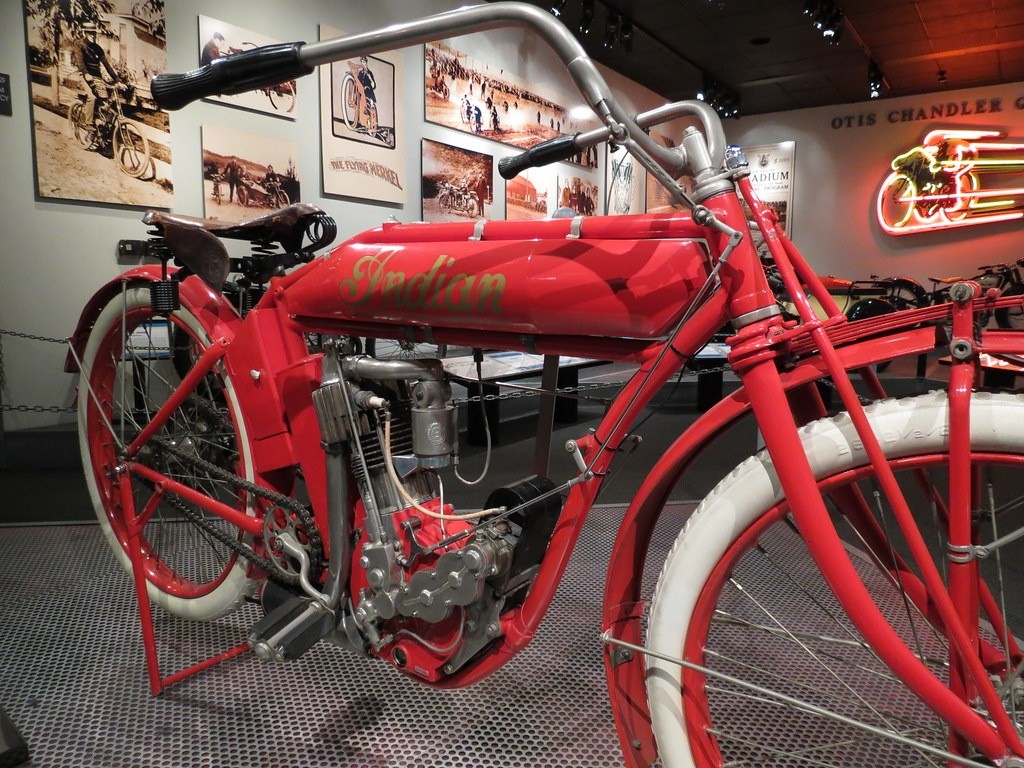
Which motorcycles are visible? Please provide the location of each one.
[842,256,1024,373]
[63,2,1024,768]
[209,174,225,204]
[438,181,480,218]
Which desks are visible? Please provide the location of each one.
[938,349,1024,390]
[829,287,889,297]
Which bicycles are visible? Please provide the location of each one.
[68,77,149,178]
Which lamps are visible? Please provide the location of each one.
[603,8,620,48]
[803,0,844,45]
[550,0,569,15]
[620,18,634,54]
[578,0,595,34]
[870,69,882,98]
[698,78,741,120]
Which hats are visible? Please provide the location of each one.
[81,23,101,32]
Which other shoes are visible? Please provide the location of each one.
[85,124,97,131]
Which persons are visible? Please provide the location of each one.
[266,164,281,210]
[73,22,128,132]
[201,32,225,67]
[476,171,487,217]
[222,156,243,202]
[562,178,595,215]
[426,48,578,133]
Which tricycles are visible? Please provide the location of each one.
[237,178,290,209]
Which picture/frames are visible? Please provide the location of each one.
[732,140,796,241]
